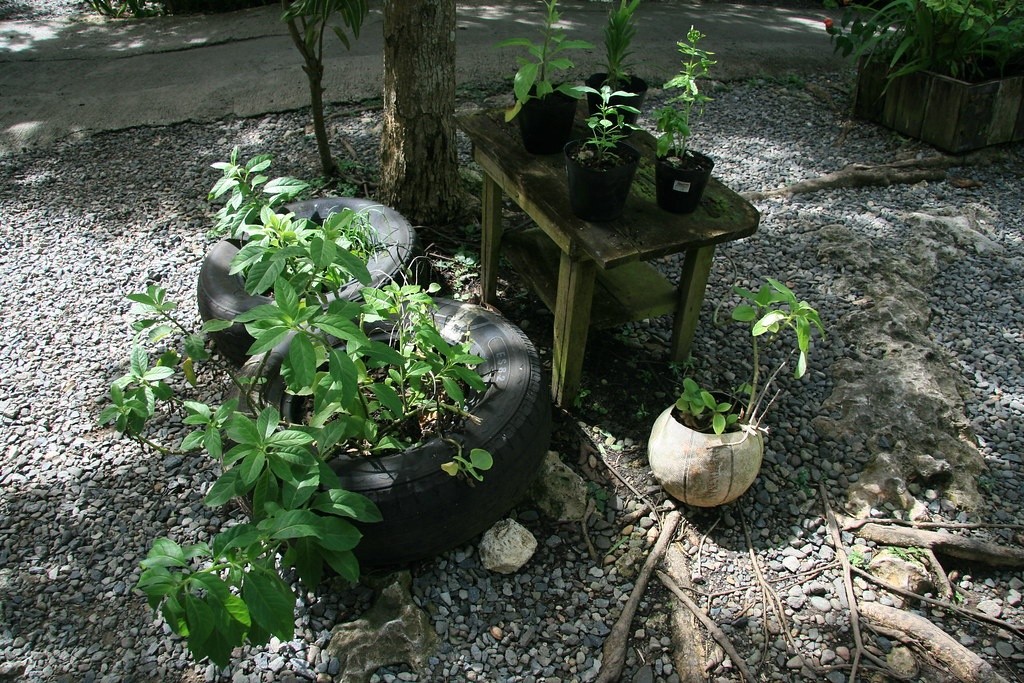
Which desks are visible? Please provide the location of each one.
[452,101,760,408]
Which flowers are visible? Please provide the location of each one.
[823,18,859,57]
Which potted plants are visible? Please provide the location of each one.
[650,277,824,511]
[95,205,552,673]
[822,1,1024,152]
[495,0,595,155]
[197,144,425,370]
[563,84,641,222]
[653,25,714,214]
[584,0,649,137]
